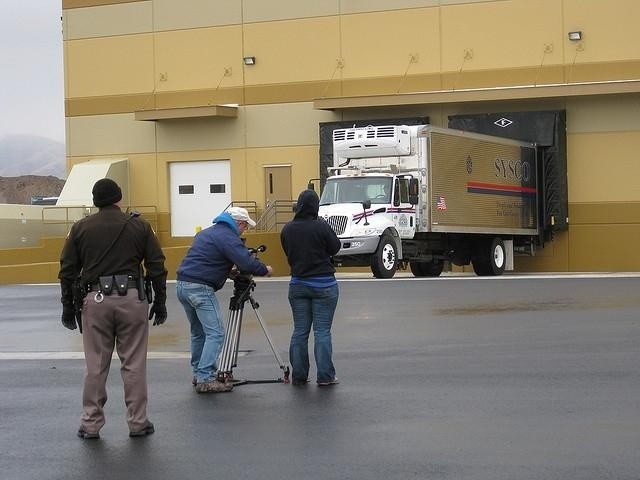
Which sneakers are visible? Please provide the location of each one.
[319,377,338,386]
[192,375,233,393]
[129,423,154,437]
[78,430,99,438]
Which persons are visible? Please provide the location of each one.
[175,206,273,394]
[281,189,341,387]
[57,178,167,440]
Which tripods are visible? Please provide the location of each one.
[216,287,290,386]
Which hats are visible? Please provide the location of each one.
[93,178,122,206]
[228,207,257,227]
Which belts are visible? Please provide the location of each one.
[90,281,137,290]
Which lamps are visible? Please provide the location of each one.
[243,56,255,65]
[568,32,581,40]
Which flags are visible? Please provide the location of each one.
[437,196,446,210]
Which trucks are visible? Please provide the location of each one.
[314,123,542,278]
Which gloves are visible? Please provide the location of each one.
[149,298,167,326]
[62,304,77,330]
